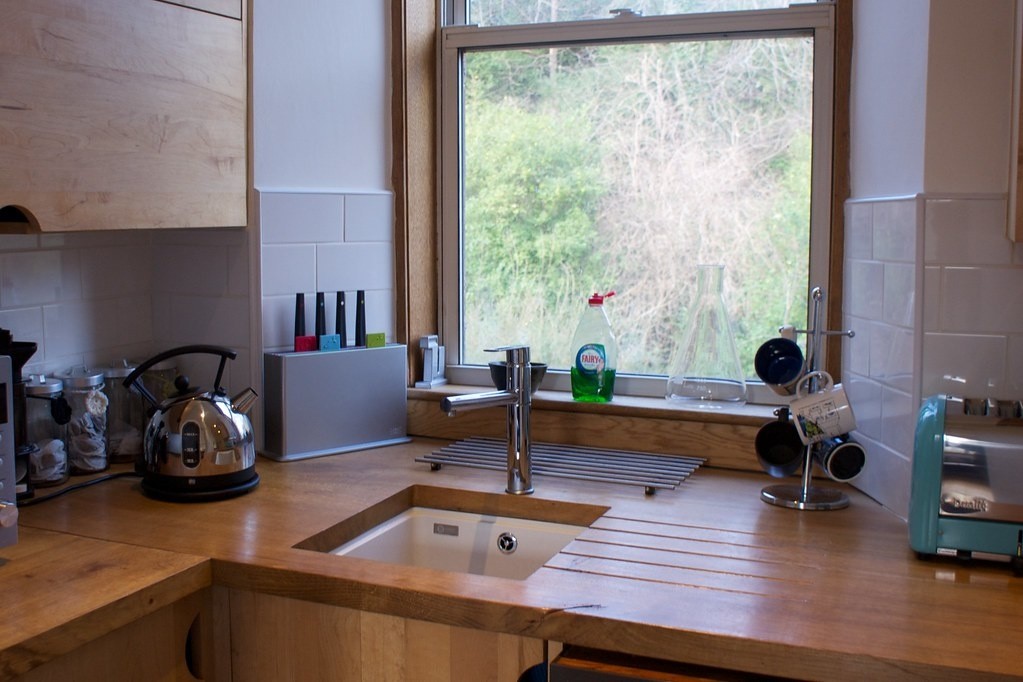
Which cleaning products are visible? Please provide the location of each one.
[570,289,618,403]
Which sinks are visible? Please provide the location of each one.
[328,505,588,580]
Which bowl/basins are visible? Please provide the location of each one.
[488,362,548,395]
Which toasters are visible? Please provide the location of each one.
[907,392,1023,572]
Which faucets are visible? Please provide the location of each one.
[440,344,536,495]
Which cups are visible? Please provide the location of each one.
[754,326,867,482]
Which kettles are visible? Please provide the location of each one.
[122,346,259,492]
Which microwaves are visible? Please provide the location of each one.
[0,356,19,548]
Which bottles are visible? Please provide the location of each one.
[665,264,748,411]
[21,357,180,488]
[570,292,618,402]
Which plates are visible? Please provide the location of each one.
[140,473,260,501]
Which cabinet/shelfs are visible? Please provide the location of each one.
[5,587,543,682]
[0,0,254,233]
